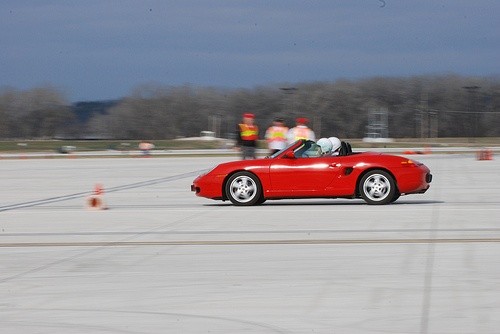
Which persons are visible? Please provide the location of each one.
[264,116,341,157]
[236,113,260,160]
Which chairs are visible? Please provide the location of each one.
[339,141,353,156]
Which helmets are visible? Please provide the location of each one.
[316,137,341,153]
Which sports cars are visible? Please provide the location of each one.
[190,138,433,206]
